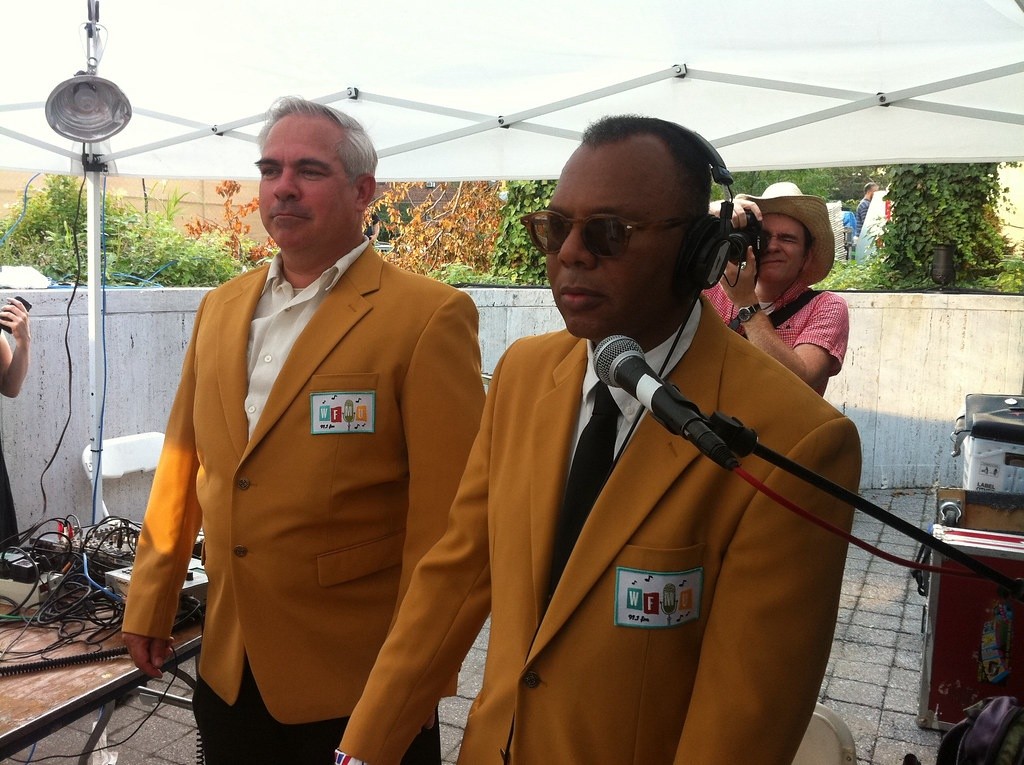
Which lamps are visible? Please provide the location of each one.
[45,71,132,144]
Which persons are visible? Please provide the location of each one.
[332,113,863,765]
[703,180,850,406]
[0,285,32,553]
[857,183,879,242]
[120,97,495,764]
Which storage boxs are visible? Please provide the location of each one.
[910,522,1024,735]
[949,394,1024,497]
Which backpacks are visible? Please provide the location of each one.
[927,692,1023,764]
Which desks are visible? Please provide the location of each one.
[0,517,203,764]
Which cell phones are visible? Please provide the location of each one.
[0,295,32,335]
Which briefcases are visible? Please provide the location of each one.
[911,517,1022,735]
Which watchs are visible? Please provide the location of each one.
[734,303,762,324]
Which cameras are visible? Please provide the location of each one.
[722,211,768,267]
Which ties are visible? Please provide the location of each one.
[546,380,629,606]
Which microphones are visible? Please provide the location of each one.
[592,333,741,472]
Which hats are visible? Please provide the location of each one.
[728,175,840,285]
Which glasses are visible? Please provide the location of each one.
[515,204,692,263]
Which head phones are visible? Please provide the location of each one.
[650,117,735,304]
